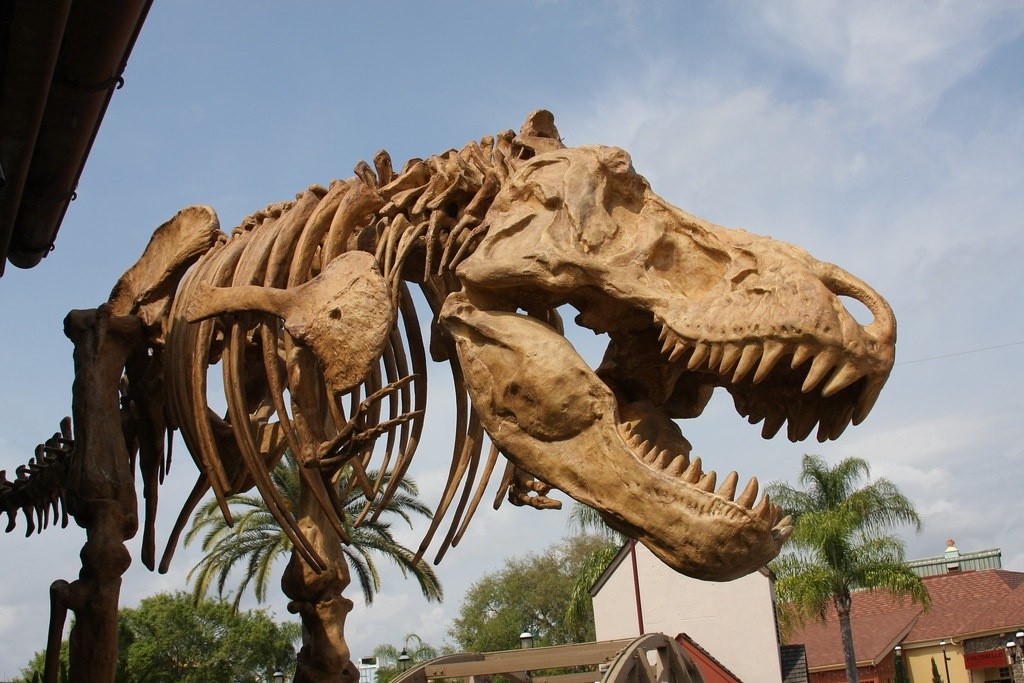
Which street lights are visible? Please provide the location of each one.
[518,630,533,677]
